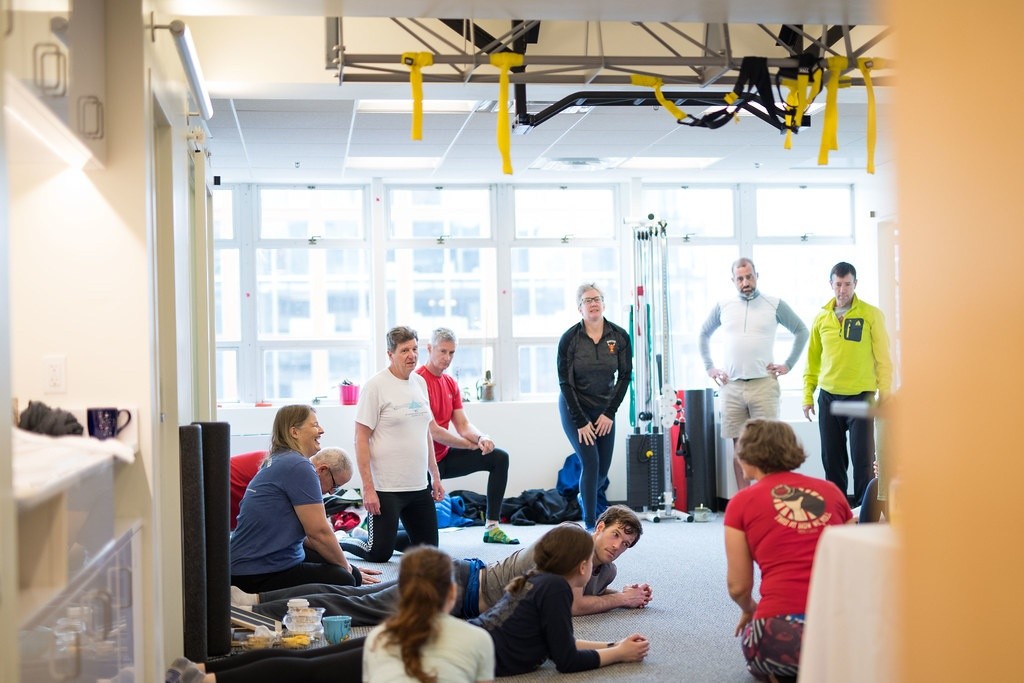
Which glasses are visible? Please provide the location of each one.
[580,296,602,306]
[329,468,338,489]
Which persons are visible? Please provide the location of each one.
[557,283,632,533]
[698,258,809,491]
[230,448,354,531]
[334,326,445,563]
[803,262,895,509]
[858,452,890,524]
[723,419,853,683]
[165,525,650,683]
[414,328,520,545]
[362,546,495,683]
[230,504,654,627]
[230,405,382,594]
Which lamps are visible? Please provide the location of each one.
[150,10,214,124]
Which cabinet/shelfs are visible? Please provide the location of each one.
[1,70,167,683]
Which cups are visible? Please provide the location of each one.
[322,615,352,645]
[694,508,709,521]
[87,408,131,439]
[287,599,310,624]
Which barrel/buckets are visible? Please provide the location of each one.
[338,385,359,405]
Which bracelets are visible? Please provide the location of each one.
[607,643,615,648]
[478,436,482,442]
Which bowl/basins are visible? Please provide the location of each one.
[283,615,321,631]
